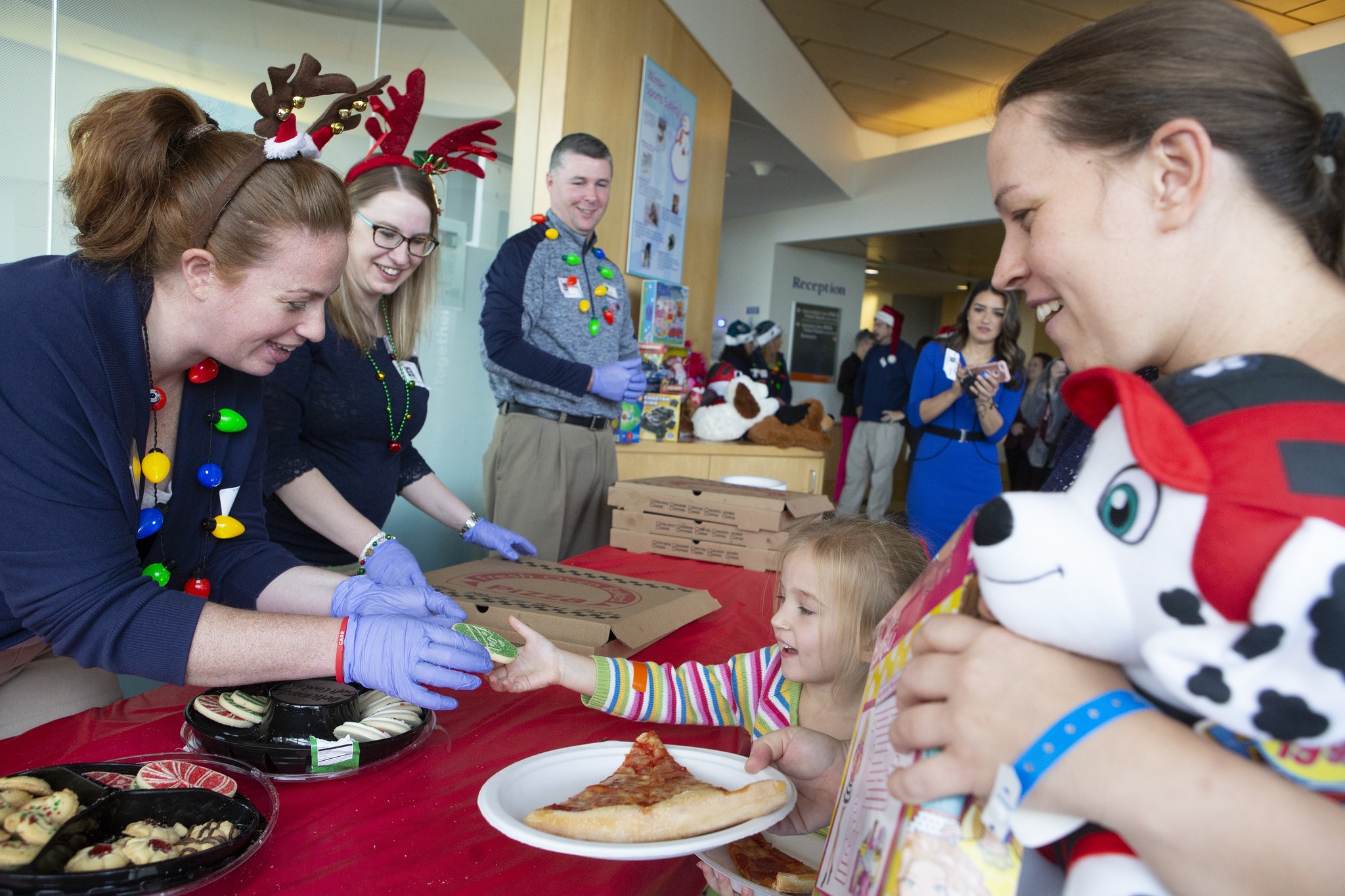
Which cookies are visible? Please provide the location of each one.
[192,689,423,743]
[451,622,519,664]
[0,760,239,873]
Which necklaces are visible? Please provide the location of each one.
[136,302,250,600]
[528,212,618,339]
[350,296,416,452]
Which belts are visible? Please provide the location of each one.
[925,424,989,444]
[497,399,613,431]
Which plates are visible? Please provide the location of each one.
[0,763,266,896]
[186,664,430,776]
[479,739,796,860]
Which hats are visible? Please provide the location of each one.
[725,319,757,347]
[934,325,958,339]
[875,304,905,364]
[755,320,782,347]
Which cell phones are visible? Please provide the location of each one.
[967,360,1011,383]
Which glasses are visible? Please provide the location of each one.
[351,209,440,257]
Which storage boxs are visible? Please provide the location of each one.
[614,278,699,446]
[415,551,728,670]
[813,496,1047,896]
[606,472,838,579]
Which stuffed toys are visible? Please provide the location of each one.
[663,341,837,455]
[895,350,1345,894]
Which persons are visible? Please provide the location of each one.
[832,329,880,504]
[482,513,929,896]
[900,279,1028,561]
[746,2,1345,896]
[480,131,648,566]
[1,86,495,740]
[255,152,540,587]
[719,319,764,387]
[833,304,919,524]
[754,320,794,408]
[904,329,1074,495]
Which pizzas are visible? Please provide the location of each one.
[525,730,789,843]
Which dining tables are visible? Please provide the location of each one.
[2,527,843,895]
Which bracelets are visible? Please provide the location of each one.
[460,510,481,539]
[356,530,397,575]
[983,690,1158,843]
[334,616,350,685]
